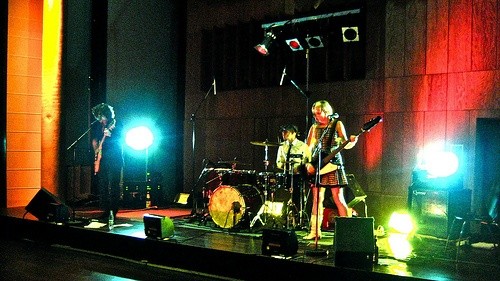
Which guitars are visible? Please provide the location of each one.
[93,119,115,173]
[296,116,383,184]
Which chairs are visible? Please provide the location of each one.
[445,193,500,262]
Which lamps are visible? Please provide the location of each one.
[143,214,174,240]
[25,188,74,224]
[334,216,379,269]
[262,229,299,257]
[254,32,277,54]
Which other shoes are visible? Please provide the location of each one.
[98,212,117,220]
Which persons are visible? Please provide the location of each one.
[275,123,311,226]
[303,100,358,239]
[91,104,123,224]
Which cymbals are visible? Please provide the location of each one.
[250,140,284,147]
[280,161,300,164]
[218,160,254,166]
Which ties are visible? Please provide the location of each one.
[286,145,292,173]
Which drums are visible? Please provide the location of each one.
[202,167,290,230]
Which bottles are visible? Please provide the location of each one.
[108,210,115,230]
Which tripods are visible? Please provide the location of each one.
[285,163,311,231]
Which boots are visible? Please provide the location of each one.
[303,214,324,240]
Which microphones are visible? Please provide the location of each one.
[91,118,100,125]
[328,113,339,118]
[214,79,216,94]
[280,66,286,85]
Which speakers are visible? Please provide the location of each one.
[143,213,175,239]
[332,217,375,268]
[26,188,72,220]
[408,187,472,240]
[261,230,299,257]
[325,173,367,209]
